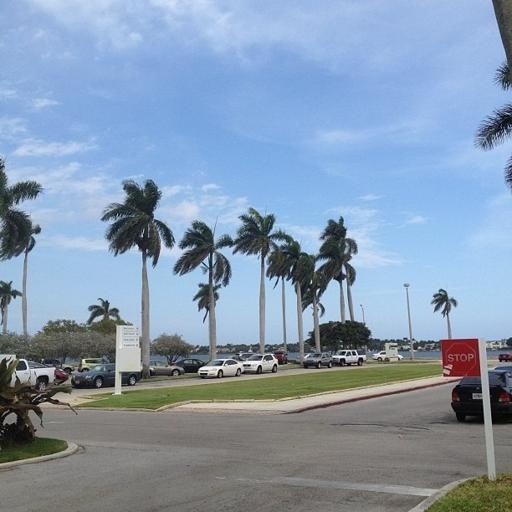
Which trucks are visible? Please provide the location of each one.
[384,342,398,353]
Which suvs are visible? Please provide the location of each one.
[243,353,279,373]
[303,352,334,369]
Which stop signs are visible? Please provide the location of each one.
[440,338,480,377]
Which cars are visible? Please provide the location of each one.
[1,353,142,392]
[372,351,403,362]
[497,352,512,362]
[492,364,512,376]
[171,358,206,373]
[239,351,255,359]
[449,369,512,422]
[198,358,244,378]
[141,359,185,376]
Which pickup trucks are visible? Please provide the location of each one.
[274,350,289,365]
[332,349,367,366]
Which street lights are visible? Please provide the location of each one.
[357,304,367,322]
[404,281,416,359]
